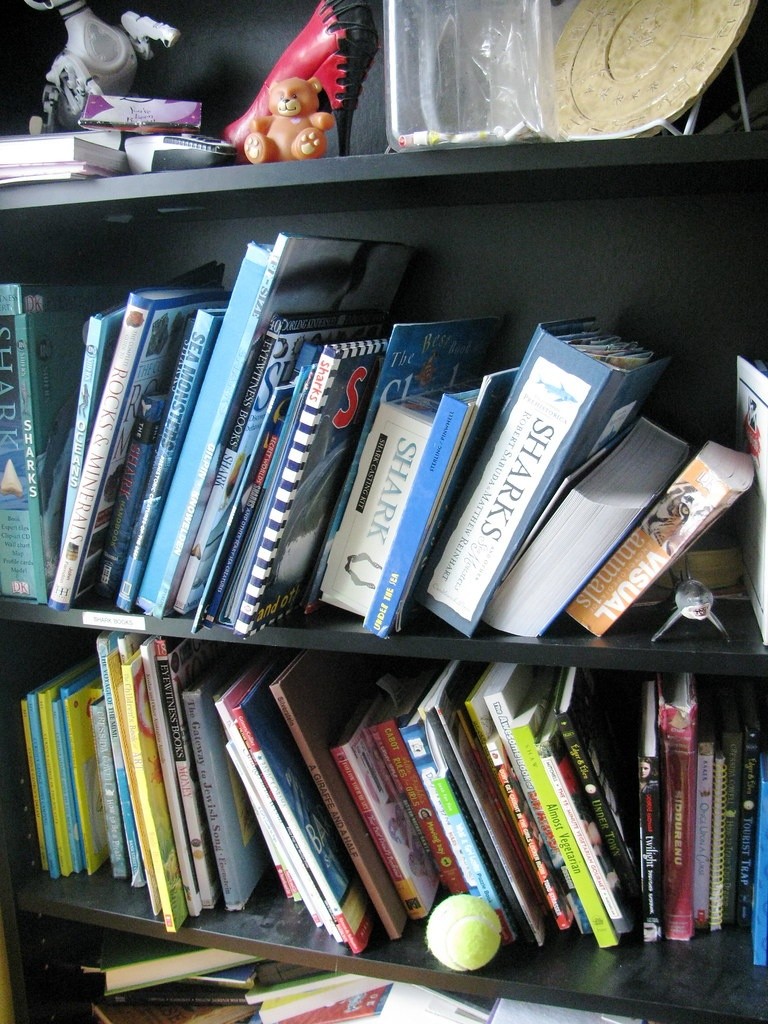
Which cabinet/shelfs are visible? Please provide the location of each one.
[0,0,768,1024]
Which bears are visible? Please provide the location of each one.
[244,76,334,165]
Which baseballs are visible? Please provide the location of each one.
[423,893,503,974]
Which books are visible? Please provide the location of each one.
[0,128,131,190]
[564,441,756,638]
[1,233,768,1024]
